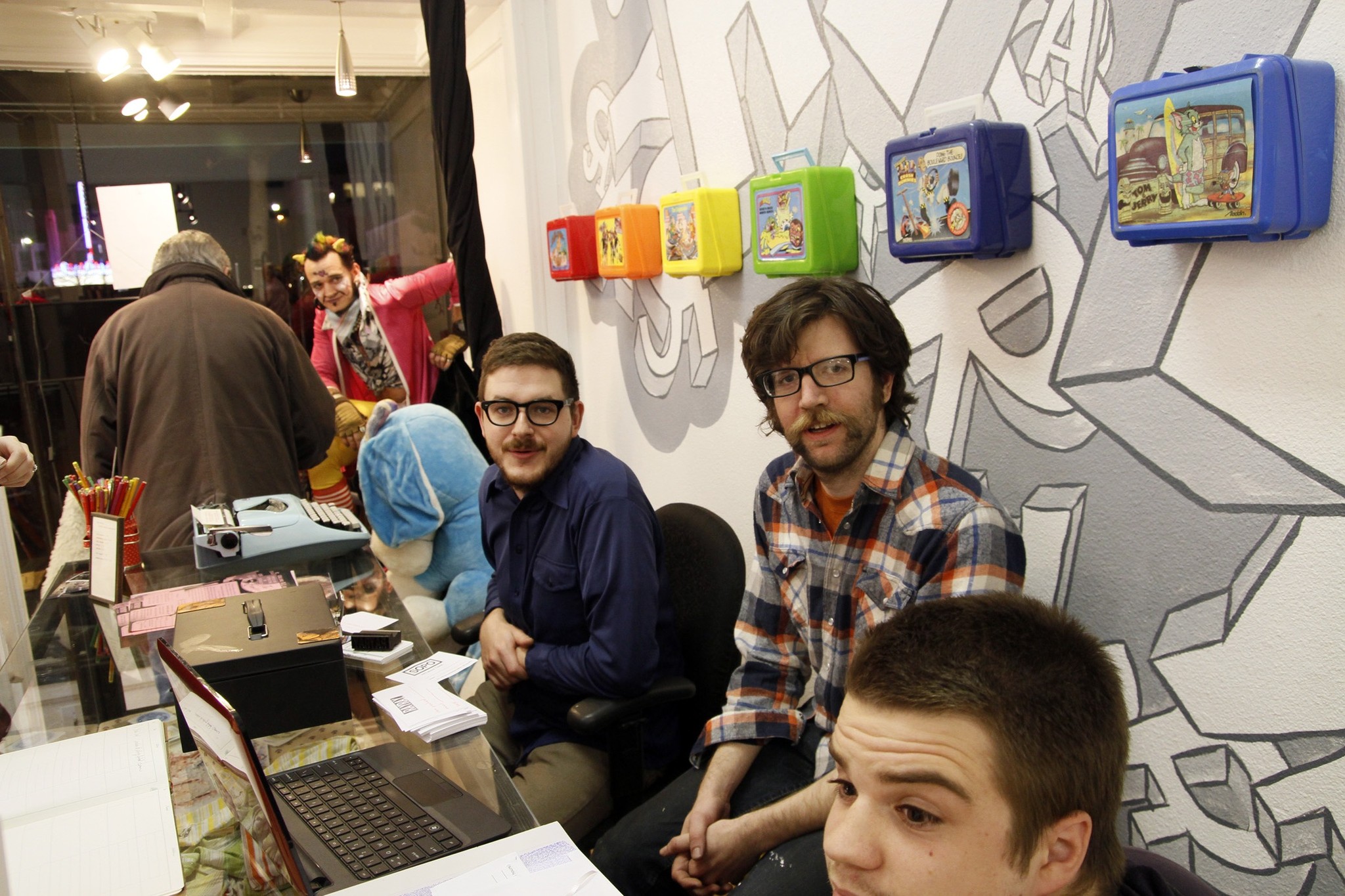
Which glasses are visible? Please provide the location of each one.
[762,352,869,399]
[480,396,575,427]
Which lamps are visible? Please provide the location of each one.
[333,0,357,97]
[287,88,314,163]
[66,4,190,123]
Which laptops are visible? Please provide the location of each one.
[155,637,513,896]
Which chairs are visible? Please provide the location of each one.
[564,503,745,849]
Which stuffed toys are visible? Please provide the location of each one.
[358,399,495,688]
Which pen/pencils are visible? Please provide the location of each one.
[62,447,147,527]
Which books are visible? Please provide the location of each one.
[0,717,185,896]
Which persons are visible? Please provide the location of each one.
[461,332,666,841]
[824,592,1226,896]
[590,279,1026,896]
[81,230,336,706]
[0,435,38,740]
[293,232,464,513]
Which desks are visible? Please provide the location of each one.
[0,544,540,896]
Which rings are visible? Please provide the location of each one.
[32,464,37,472]
